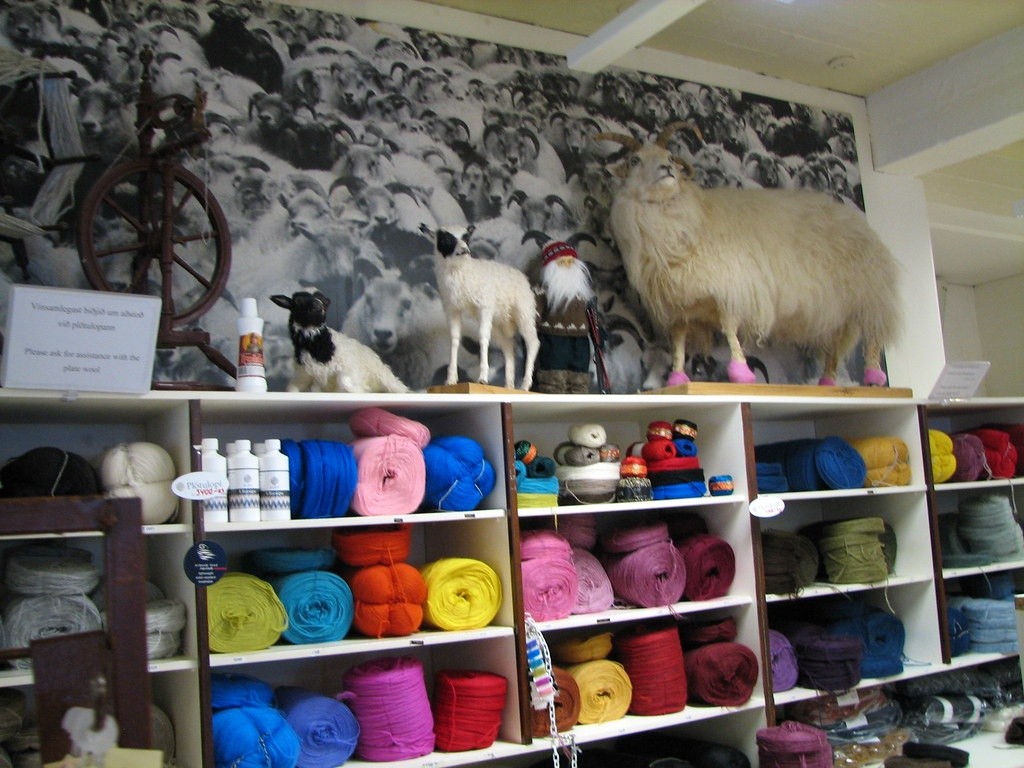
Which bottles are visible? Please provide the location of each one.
[225,443,237,458]
[259,439,291,521]
[234,297,268,392]
[227,439,261,522]
[201,438,228,524]
[253,442,266,458]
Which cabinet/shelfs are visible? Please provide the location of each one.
[0,392,1024,768]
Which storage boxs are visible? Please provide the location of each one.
[1,284,163,395]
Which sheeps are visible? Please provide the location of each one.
[417,222,543,395]
[269,286,409,401]
[591,121,903,385]
